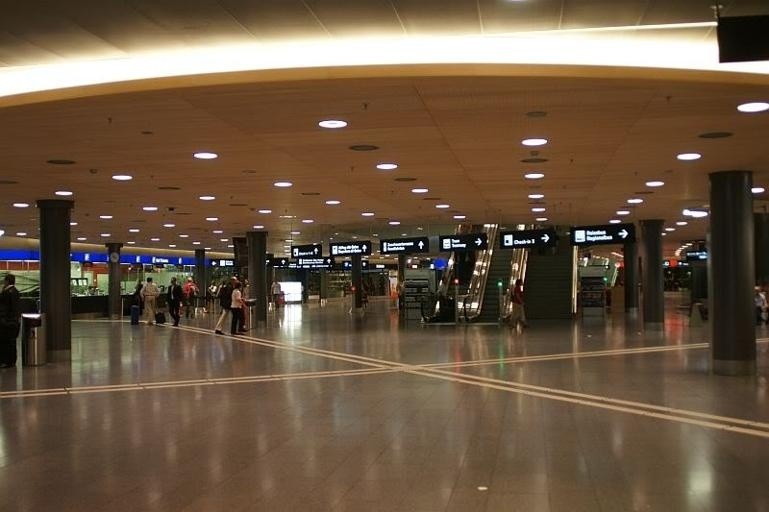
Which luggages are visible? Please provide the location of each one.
[155,311,165,323]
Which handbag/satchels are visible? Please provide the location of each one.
[189,285,199,297]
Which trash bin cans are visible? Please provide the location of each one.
[21,311,47,369]
[244,298,257,331]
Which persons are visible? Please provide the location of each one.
[754,285,768,328]
[508,278,529,328]
[360,278,370,309]
[130,274,282,336]
[1,274,22,370]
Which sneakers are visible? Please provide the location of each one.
[214,330,225,335]
[232,328,248,334]
